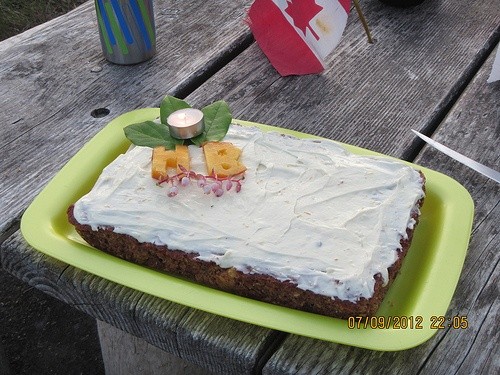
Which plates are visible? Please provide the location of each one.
[19,105,474,351]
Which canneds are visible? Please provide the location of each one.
[94,0,157,65]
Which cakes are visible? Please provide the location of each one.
[67,110,426,323]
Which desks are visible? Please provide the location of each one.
[1,1,500,374]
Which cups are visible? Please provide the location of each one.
[95,0,157,66]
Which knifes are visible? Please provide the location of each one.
[411,129,500,185]
